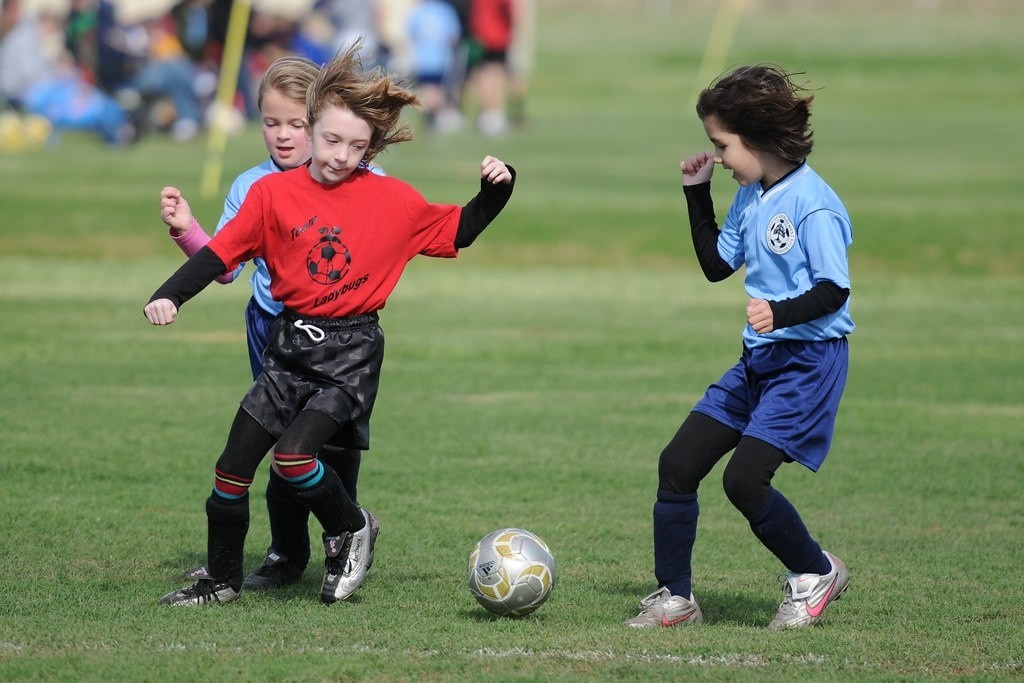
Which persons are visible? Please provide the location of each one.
[145,43,517,607]
[0,0,534,142]
[622,65,855,628]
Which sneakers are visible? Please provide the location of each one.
[158,566,245,612]
[319,506,380,605]
[244,547,310,595]
[767,549,853,635]
[621,584,702,631]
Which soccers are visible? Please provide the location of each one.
[465,527,558,618]
